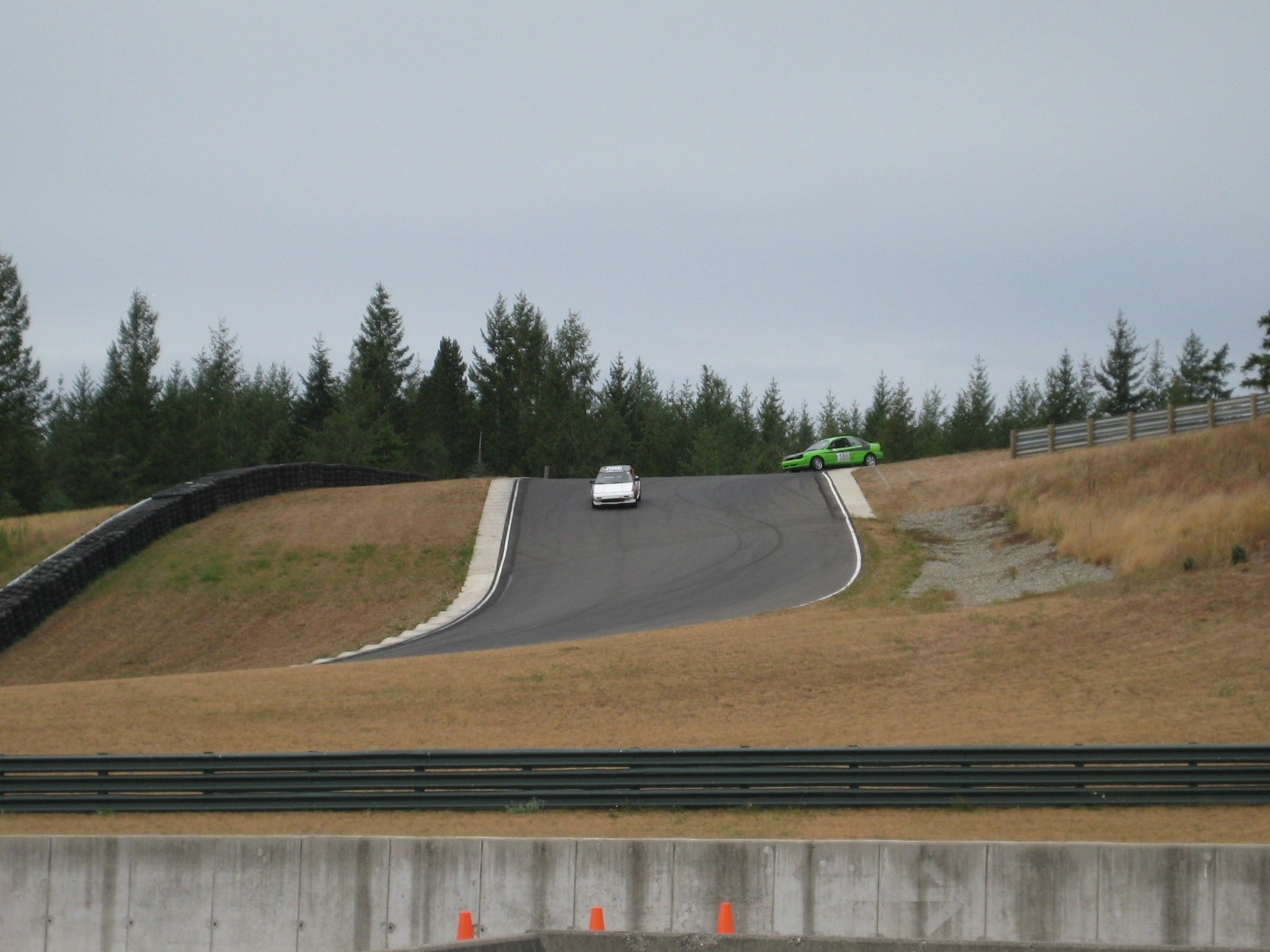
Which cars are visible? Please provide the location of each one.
[590,464,641,508]
[782,435,883,472]
